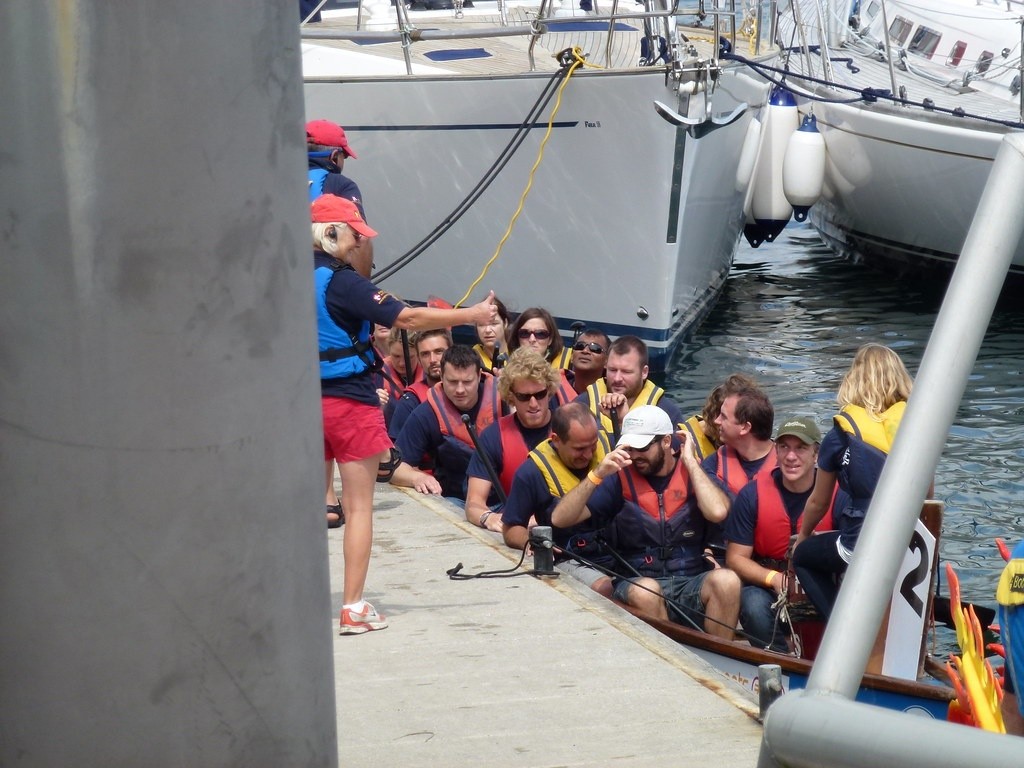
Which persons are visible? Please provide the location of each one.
[571,336,686,451]
[552,405,741,640]
[472,298,511,374]
[465,345,561,532]
[498,307,574,371]
[788,342,913,617]
[311,193,497,633]
[701,374,778,569]
[676,384,724,460]
[722,417,839,657]
[553,328,612,407]
[502,402,616,596]
[374,324,454,439]
[306,120,403,528]
[389,344,517,508]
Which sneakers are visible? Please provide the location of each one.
[338,600,389,635]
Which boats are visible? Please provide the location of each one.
[299,1,1024,379]
[616,590,1024,730]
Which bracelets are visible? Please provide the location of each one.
[765,570,778,587]
[588,469,603,484]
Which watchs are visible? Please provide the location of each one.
[480,512,492,527]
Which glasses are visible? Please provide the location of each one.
[574,341,607,354]
[346,229,362,242]
[635,440,657,452]
[518,329,550,339]
[510,389,548,402]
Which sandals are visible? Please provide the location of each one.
[376,444,402,482]
[326,500,346,528]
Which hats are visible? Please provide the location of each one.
[615,405,674,449]
[306,119,357,160]
[311,193,378,238]
[775,417,823,445]
